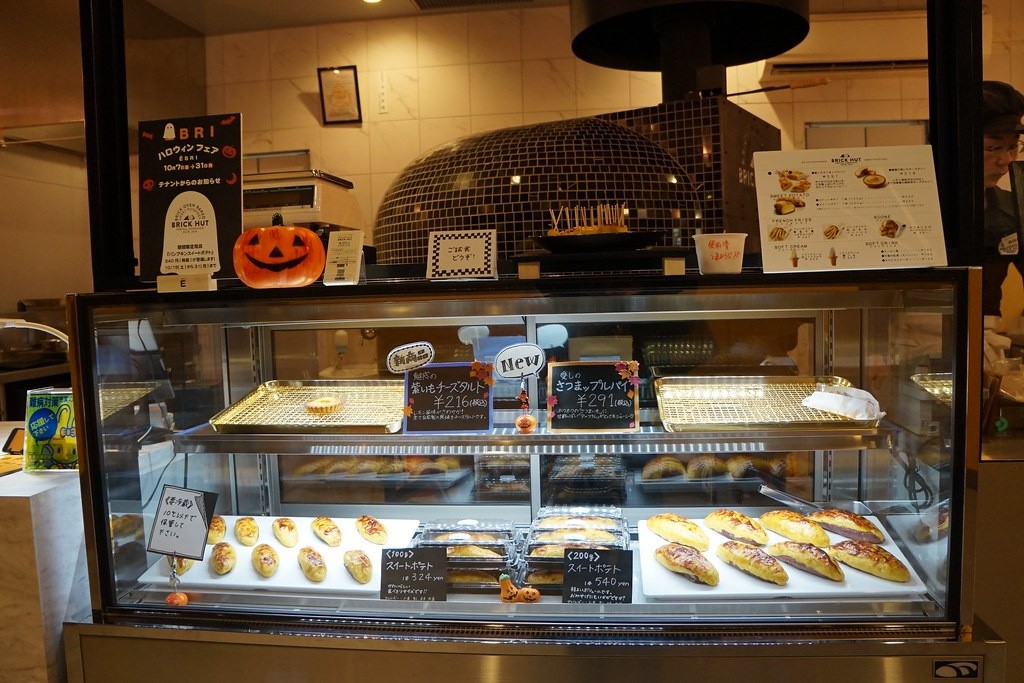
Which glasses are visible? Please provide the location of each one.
[983,139,1024,154]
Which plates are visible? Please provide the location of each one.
[529,230,666,254]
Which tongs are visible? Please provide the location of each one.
[759,483,822,515]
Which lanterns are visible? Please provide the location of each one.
[233,212,326,289]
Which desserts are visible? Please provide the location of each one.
[305,396,340,413]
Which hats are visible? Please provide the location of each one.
[982,80,1024,135]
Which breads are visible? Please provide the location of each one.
[168,507,913,585]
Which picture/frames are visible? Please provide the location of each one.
[317,65,363,126]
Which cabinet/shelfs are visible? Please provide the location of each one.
[66,266,984,651]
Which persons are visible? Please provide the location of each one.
[959,79,1024,423]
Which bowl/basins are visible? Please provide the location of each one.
[691,233,749,274]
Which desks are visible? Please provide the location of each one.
[0,421,117,683]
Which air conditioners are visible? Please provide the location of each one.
[757,8,993,83]
